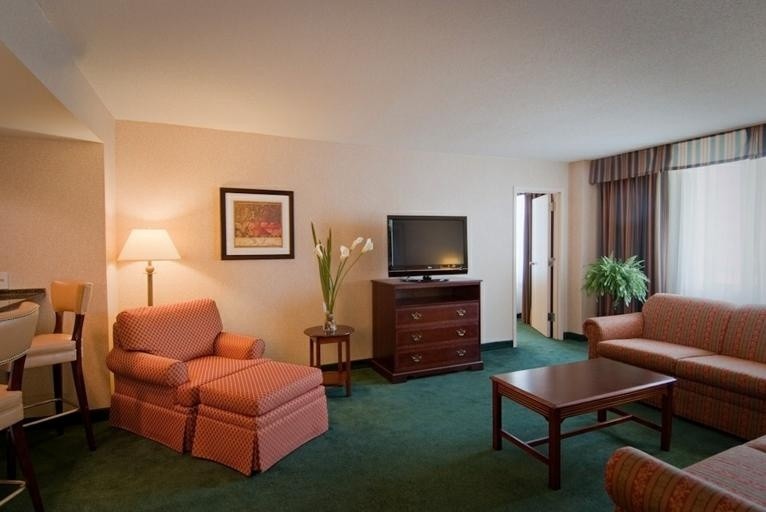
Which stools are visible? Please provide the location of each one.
[190,360,329,477]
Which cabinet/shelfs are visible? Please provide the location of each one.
[371,279,484,383]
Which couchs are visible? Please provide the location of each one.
[582,293,766,440]
[605,434,766,512]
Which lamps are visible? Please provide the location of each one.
[117,228,180,306]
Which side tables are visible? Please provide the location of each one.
[304,325,355,396]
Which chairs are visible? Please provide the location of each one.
[0,302,45,511]
[106,299,271,452]
[5,280,95,454]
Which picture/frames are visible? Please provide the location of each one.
[220,187,295,259]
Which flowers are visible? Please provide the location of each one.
[309,222,373,314]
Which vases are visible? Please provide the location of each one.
[322,302,337,331]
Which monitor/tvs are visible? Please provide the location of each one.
[387,214,469,282]
